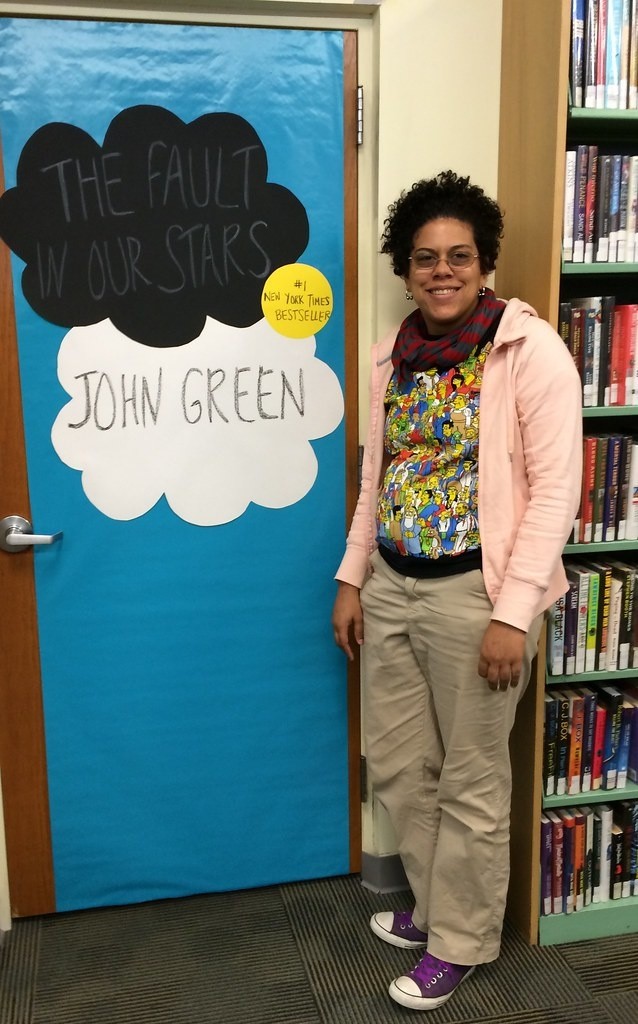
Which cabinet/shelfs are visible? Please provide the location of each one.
[493,0,638,946]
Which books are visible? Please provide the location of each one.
[541,0,638,917]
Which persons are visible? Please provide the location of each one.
[331,168,583,1010]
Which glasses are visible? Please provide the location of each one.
[407,252,480,269]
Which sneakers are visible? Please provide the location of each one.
[370,911,428,949]
[388,951,476,1010]
